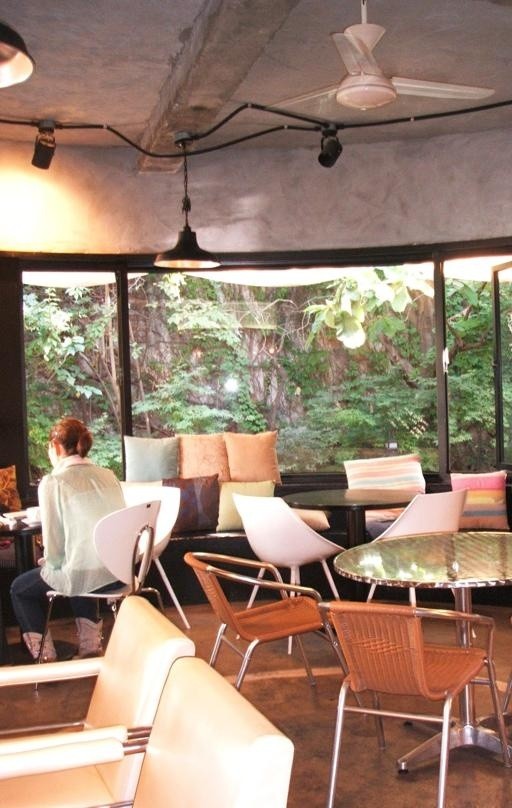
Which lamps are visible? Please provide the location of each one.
[0,23,36,89]
[317,122,343,166]
[31,119,57,170]
[153,138,222,268]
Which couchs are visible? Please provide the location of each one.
[0,470,444,628]
[419,483,512,607]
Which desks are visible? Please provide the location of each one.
[1,519,78,662]
[332,530,512,773]
[282,490,423,600]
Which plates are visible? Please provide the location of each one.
[3,512,26,518]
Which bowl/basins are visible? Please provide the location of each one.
[27,507,40,518]
[21,518,42,527]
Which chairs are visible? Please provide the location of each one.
[0,655,294,808]
[318,599,512,807]
[0,595,195,808]
[230,491,340,657]
[121,486,191,630]
[182,551,368,718]
[366,489,477,643]
[33,499,161,691]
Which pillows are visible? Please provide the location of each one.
[0,465,23,512]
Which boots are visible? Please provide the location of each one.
[71,616,105,660]
[22,627,57,662]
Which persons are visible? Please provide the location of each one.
[10,416,127,665]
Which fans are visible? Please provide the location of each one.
[262,0,495,111]
